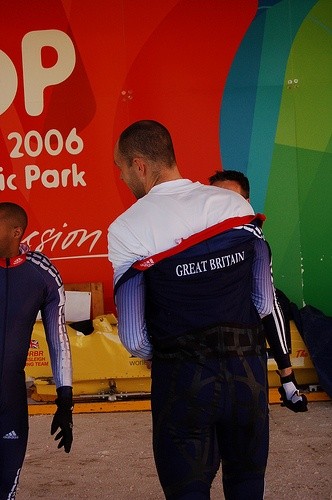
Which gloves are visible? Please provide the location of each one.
[50,397,75,453]
[274,369,308,413]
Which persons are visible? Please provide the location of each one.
[209,171,251,202]
[107,120,308,500]
[0,202,74,500]
